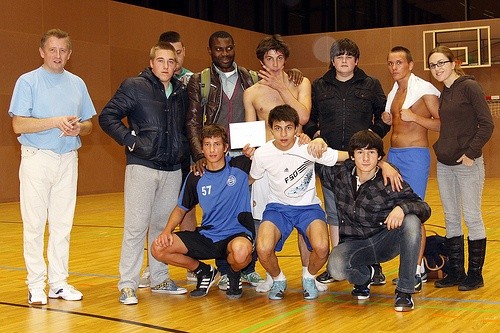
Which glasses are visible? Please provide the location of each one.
[429,59,453,70]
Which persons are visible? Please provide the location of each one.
[307,130,432,312]
[248,104,403,300]
[138,31,198,288]
[381,46,441,293]
[428,45,494,291]
[186,31,305,291]
[243,34,329,293]
[151,123,311,301]
[8,28,97,305]
[99,42,189,305]
[302,38,391,284]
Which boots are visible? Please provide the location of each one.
[434,235,466,287]
[458,237,487,291]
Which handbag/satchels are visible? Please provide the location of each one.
[422,235,452,281]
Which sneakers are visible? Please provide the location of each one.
[239,272,265,286]
[28,287,47,305]
[217,272,230,290]
[352,265,375,300]
[269,279,288,300]
[393,288,412,311]
[370,264,385,284]
[226,270,243,299]
[391,270,427,285]
[190,263,221,298]
[137,266,151,287]
[152,278,188,293]
[303,278,318,299]
[316,271,335,283]
[186,269,198,282]
[412,276,423,293]
[120,287,138,304]
[48,285,83,300]
